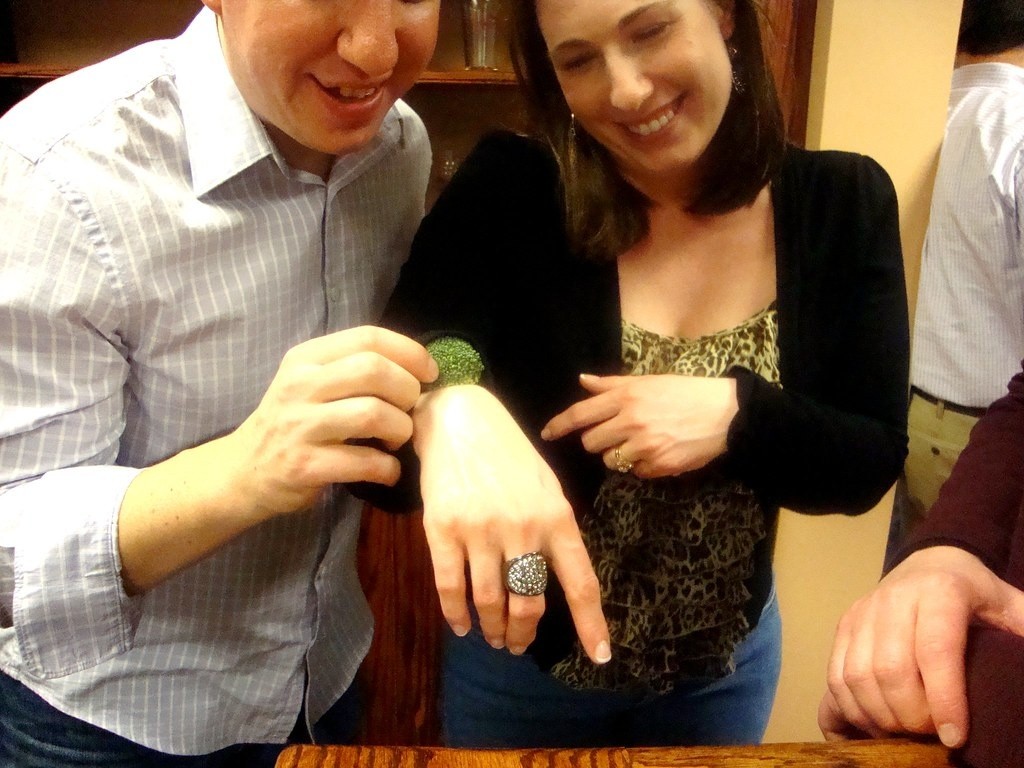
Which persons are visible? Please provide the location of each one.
[817,363,1024,768]
[904,0,1023,520]
[334,2,910,750]
[1,0,440,768]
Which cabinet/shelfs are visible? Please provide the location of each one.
[0,1,817,746]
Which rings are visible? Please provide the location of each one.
[615,444,634,472]
[501,551,548,596]
